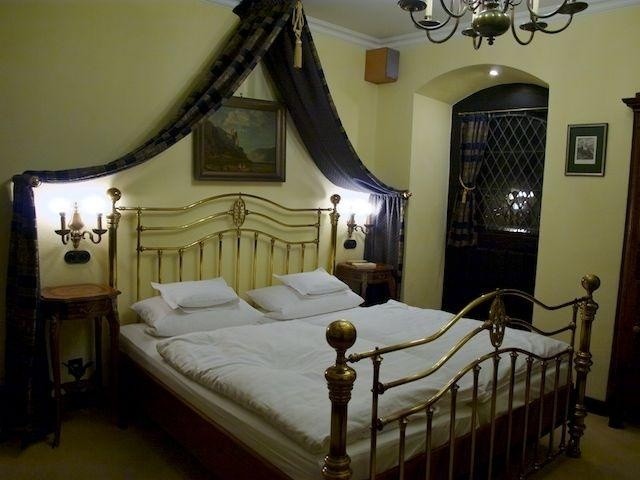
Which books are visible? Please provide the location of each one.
[346,260,377,268]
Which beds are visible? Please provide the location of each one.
[108,188,601,480]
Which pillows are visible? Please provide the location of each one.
[150,276,240,314]
[246,284,366,320]
[129,295,267,338]
[272,267,350,299]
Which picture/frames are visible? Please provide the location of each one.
[193,96,287,182]
[565,123,608,177]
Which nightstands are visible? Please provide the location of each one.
[36,283,128,446]
[336,261,397,307]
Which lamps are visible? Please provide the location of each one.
[54,202,107,249]
[347,213,375,238]
[397,0,588,52]
